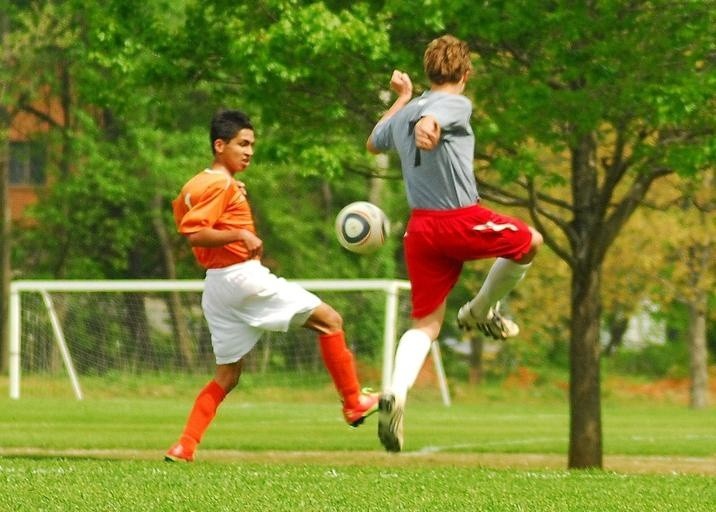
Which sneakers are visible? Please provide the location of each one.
[458,301,519,340]
[343,392,381,427]
[164,439,194,462]
[377,392,404,450]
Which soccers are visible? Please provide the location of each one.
[335,201,391,253]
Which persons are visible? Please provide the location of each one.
[366,33,544,454]
[162,109,383,463]
